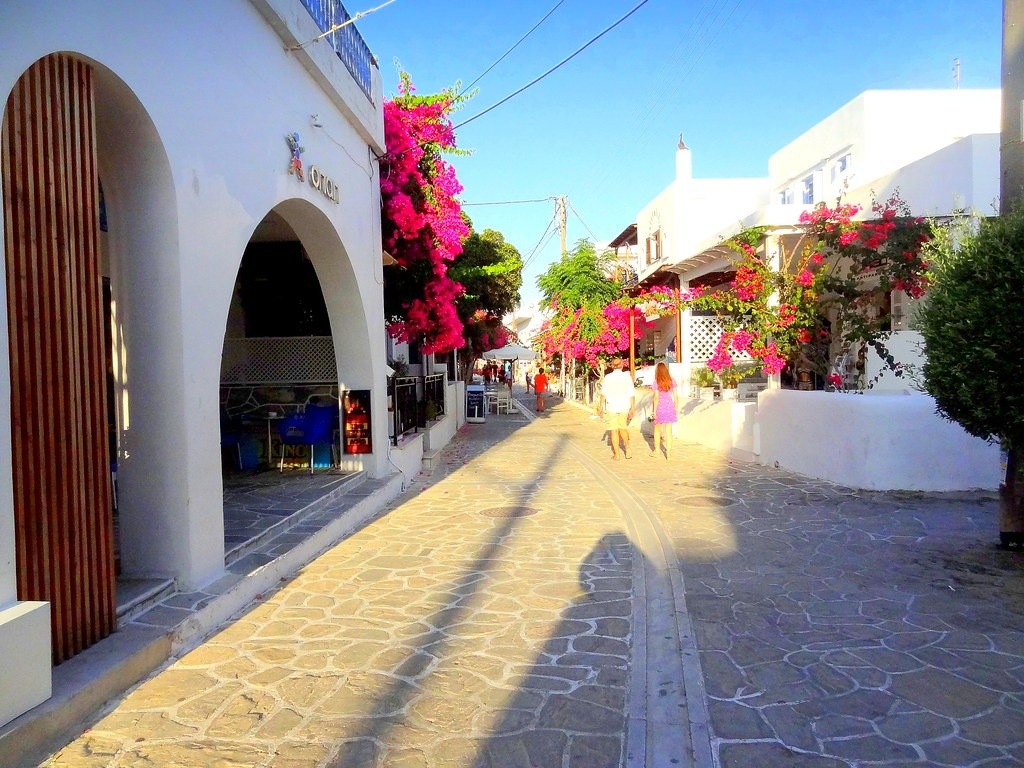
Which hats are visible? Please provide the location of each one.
[612,358,623,368]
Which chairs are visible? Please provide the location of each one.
[277,402,341,473]
[484,382,512,415]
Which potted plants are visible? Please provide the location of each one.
[719,363,740,401]
[696,366,716,401]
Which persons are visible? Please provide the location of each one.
[598,357,635,460]
[534,368,549,412]
[481,358,512,388]
[648,363,678,460]
[525,372,535,393]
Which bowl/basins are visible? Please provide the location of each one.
[268,412,277,417]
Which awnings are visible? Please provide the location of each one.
[483,343,541,360]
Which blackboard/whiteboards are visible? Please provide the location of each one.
[465,389,485,419]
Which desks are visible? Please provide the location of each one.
[264,416,290,463]
[484,391,498,412]
